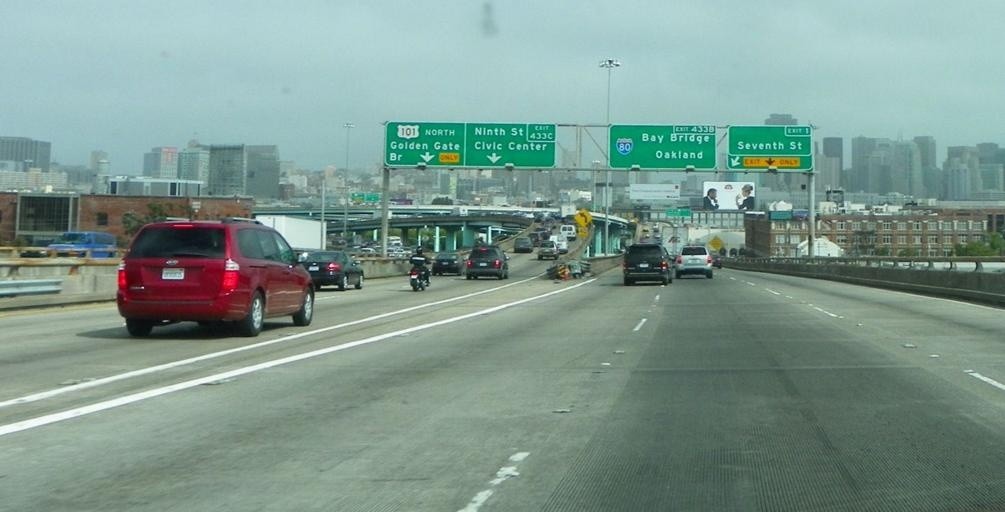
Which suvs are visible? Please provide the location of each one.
[467,245,510,280]
[675,245,714,279]
[621,243,674,286]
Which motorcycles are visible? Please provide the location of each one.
[407,265,431,292]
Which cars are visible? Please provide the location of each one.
[708,249,722,268]
[432,252,466,275]
[297,251,364,291]
[640,221,679,243]
[21,239,52,257]
[511,211,577,261]
[332,235,414,260]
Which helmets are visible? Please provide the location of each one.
[416,245,424,254]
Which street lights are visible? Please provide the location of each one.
[342,121,356,234]
[598,59,622,256]
[591,158,601,213]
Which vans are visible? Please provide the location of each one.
[116,215,316,337]
[47,231,117,260]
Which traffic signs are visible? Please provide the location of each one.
[728,124,814,172]
[351,193,363,201]
[363,192,379,202]
[665,208,691,216]
[608,123,718,174]
[465,123,558,170]
[385,119,466,169]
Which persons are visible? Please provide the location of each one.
[410,246,430,281]
[736,185,754,210]
[704,188,721,209]
